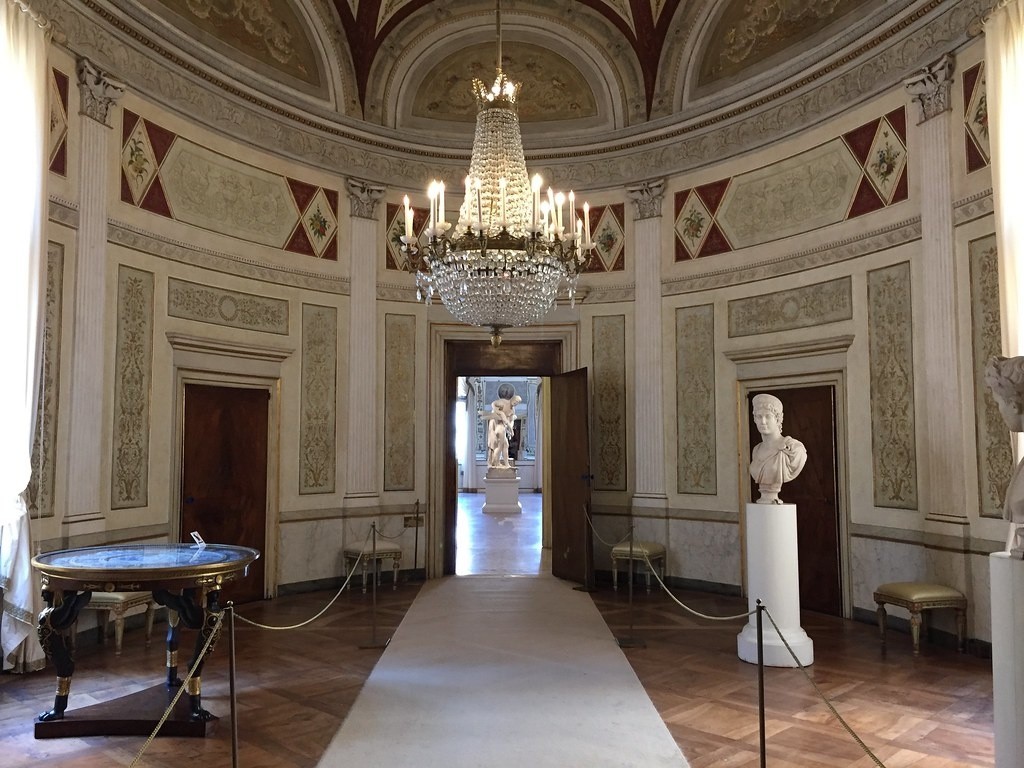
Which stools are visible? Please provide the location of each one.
[340,541,403,594]
[66,591,154,656]
[612,541,666,595]
[873,583,965,656]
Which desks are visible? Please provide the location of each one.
[31,544,264,740]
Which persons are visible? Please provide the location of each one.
[986,355,1024,559]
[749,394,806,503]
[487,396,522,467]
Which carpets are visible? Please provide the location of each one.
[313,577,690,766]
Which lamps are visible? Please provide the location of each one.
[401,1,598,344]
